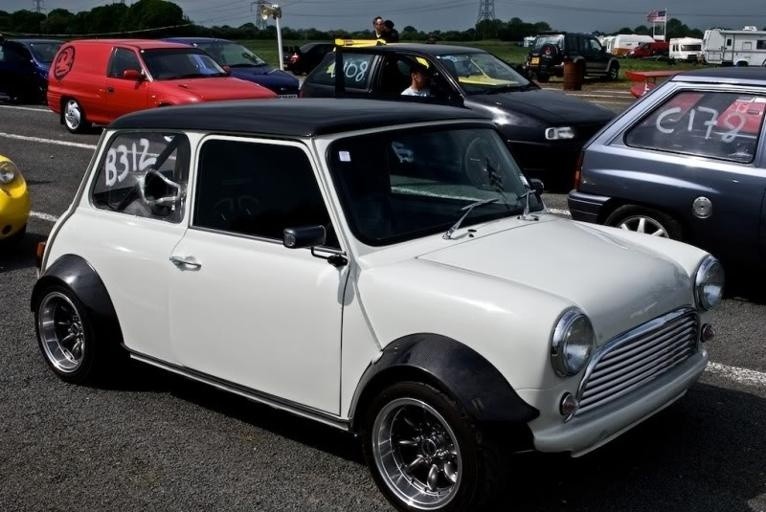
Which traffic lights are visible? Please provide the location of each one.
[288,44,303,75]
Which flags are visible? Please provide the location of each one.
[647,10,666,22]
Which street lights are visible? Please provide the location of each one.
[258,3,289,72]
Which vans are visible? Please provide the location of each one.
[42,38,279,135]
[296,43,619,191]
[561,62,766,248]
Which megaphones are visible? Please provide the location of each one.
[260,5,277,20]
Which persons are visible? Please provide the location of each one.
[400,61,437,99]
[372,15,393,46]
[383,20,398,41]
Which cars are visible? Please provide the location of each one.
[26,96,729,512]
[623,64,766,157]
[0,146,32,248]
